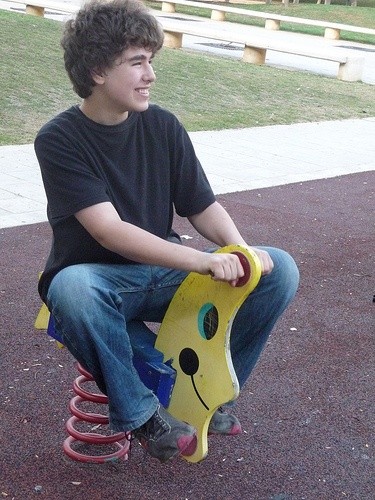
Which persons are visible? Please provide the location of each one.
[33,0,301,465]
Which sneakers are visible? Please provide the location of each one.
[126,404,196,460]
[209,407,240,435]
